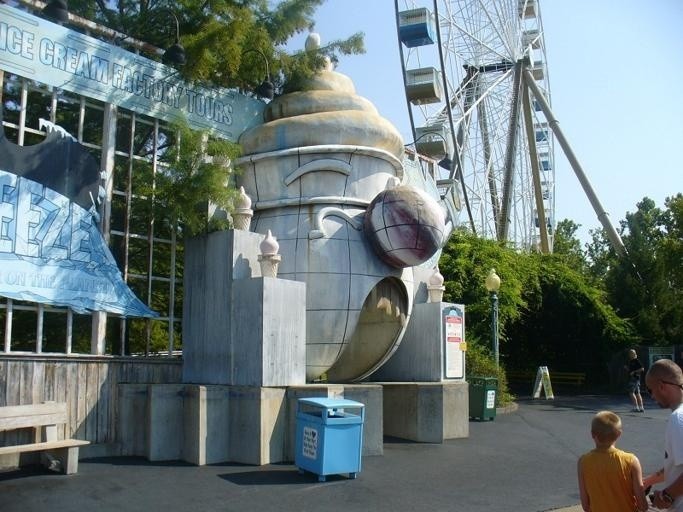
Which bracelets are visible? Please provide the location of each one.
[662,489,673,505]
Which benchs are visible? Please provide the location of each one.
[511,370,585,392]
[0,401,91,475]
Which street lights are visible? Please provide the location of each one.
[487,268,500,364]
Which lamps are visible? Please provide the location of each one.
[141,9,184,64]
[242,49,273,100]
[42,0,70,24]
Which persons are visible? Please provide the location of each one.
[638,359,682,511]
[623,349,646,413]
[577,409,649,512]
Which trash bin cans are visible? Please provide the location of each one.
[466,377,498,422]
[295,397,365,482]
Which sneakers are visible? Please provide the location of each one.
[631,407,646,413]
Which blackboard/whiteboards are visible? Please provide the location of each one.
[532,365,554,400]
